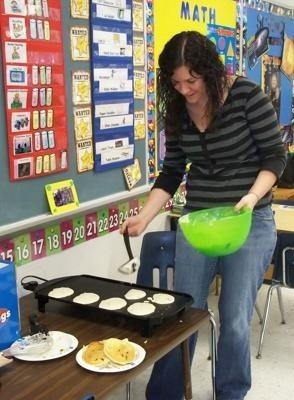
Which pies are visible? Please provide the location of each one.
[82,341,110,367]
[99,297,127,310]
[125,289,146,300]
[127,303,156,316]
[147,293,174,304]
[103,338,137,365]
[48,287,74,299]
[73,292,100,305]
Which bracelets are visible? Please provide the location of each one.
[249,190,260,202]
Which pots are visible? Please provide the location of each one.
[24,273,195,338]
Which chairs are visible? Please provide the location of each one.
[126,230,216,399]
[255,233,294,357]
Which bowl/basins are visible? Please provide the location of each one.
[176,205,252,258]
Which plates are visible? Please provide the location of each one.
[10,330,147,374]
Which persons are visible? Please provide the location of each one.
[266,71,279,116]
[120,29,287,399]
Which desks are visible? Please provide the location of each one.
[0,293,218,400]
